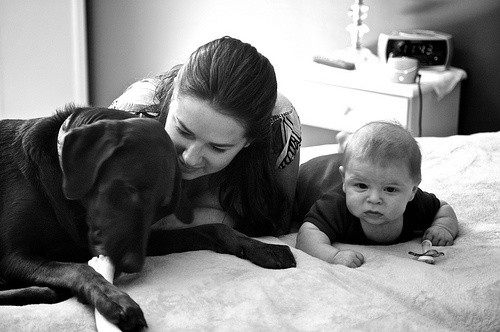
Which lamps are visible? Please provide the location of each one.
[345,0,370,56]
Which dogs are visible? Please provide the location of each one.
[0,102,297,332]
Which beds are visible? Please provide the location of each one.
[1,128,500,331]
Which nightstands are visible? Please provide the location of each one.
[293,67,467,148]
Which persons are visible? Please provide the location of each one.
[105,36,303,236]
[293,121,459,267]
